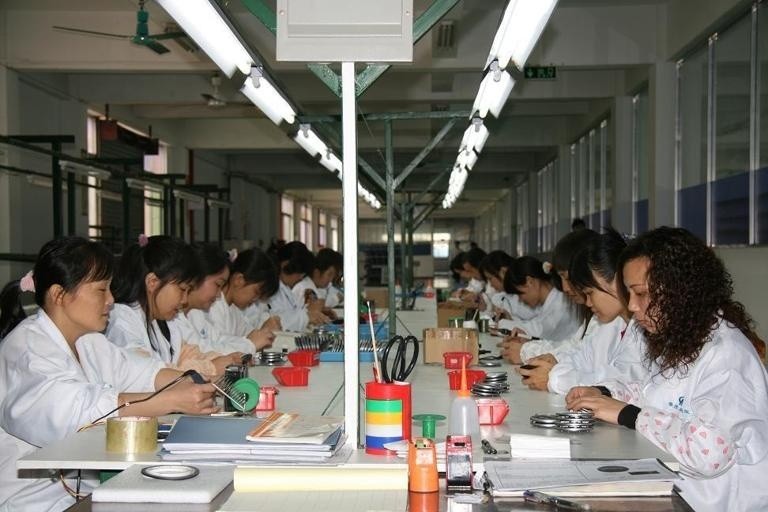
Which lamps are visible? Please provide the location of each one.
[22,157,236,212]
[157,0,382,213]
[437,0,558,211]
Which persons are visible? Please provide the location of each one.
[448,217,768,511]
[1,235,343,511]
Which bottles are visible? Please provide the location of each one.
[449,354,482,451]
[463,321,478,330]
[449,316,463,327]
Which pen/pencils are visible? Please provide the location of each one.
[266,303,274,318]
[482,440,497,454]
[523,489,590,512]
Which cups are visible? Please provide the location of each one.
[363,380,412,455]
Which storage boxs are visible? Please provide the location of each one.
[364,290,389,309]
[435,301,466,327]
[422,326,479,367]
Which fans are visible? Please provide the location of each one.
[50,0,184,55]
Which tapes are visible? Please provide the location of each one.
[106,414,158,454]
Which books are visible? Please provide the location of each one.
[383,439,447,473]
[216,467,408,512]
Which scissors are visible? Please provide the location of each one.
[381,335,419,383]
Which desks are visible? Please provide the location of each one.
[59,485,695,511]
[17,287,684,482]
